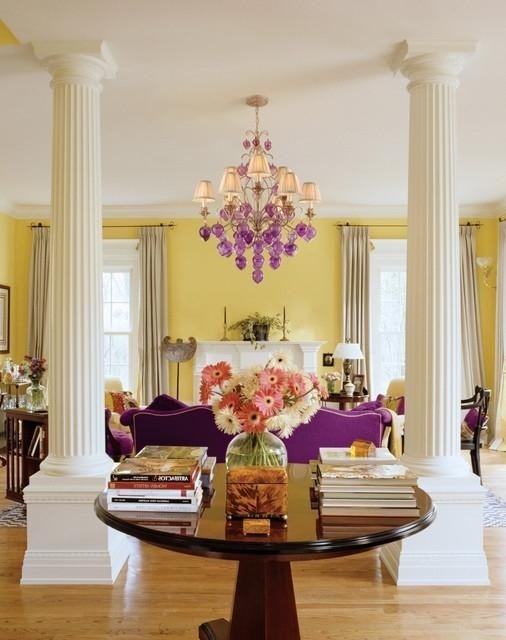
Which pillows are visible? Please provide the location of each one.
[376,394,403,415]
[460,408,489,442]
[145,393,187,410]
[104,391,140,413]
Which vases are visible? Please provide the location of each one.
[224,430,294,466]
[26,376,47,410]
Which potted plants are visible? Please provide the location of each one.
[228,311,290,344]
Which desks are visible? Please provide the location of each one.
[319,389,369,410]
[95,460,436,639]
[2,405,48,506]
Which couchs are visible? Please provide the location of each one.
[105,407,383,465]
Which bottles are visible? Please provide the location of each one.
[2,359,21,383]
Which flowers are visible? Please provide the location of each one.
[197,351,329,467]
[23,354,48,401]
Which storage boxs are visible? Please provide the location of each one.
[223,469,289,521]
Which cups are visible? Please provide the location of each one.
[344,383,355,397]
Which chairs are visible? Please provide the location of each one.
[461,386,490,484]
[104,378,140,414]
[380,376,406,458]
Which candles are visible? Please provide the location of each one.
[223,306,226,324]
[282,306,286,324]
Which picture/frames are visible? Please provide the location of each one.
[0,283,11,354]
[352,374,365,396]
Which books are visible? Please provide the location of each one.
[106,445,217,512]
[307,444,421,518]
[317,515,419,539]
[108,512,215,536]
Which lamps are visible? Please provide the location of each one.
[193,95,322,284]
[332,337,364,394]
[476,256,496,290]
